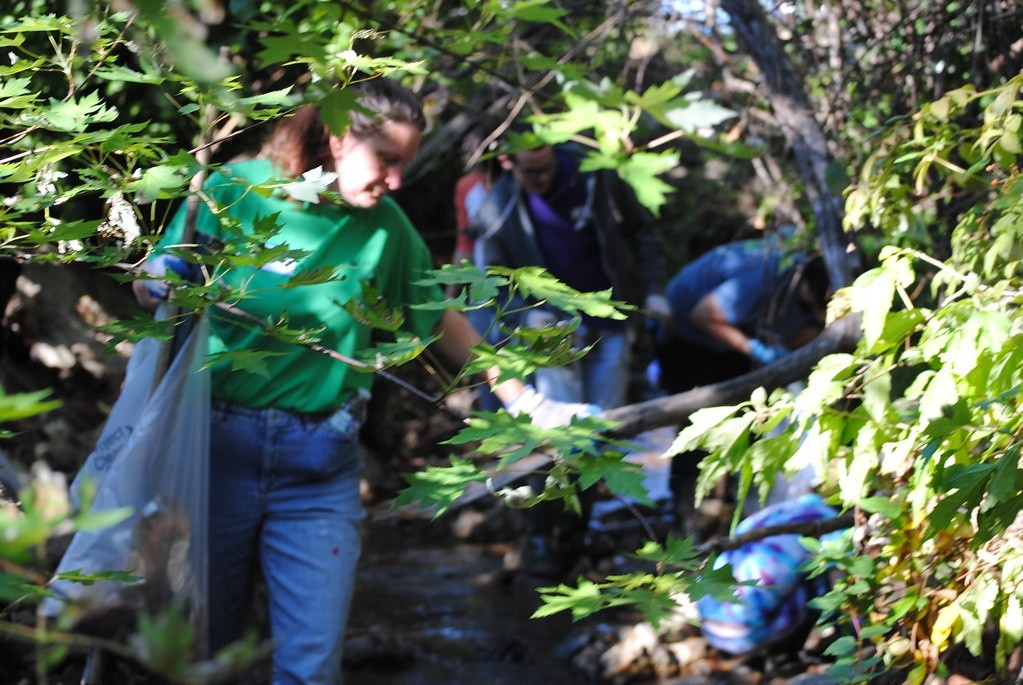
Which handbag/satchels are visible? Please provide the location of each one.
[34,295,211,685]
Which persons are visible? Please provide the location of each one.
[82,76,858,685]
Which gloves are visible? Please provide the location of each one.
[503,386,602,435]
[749,339,790,366]
